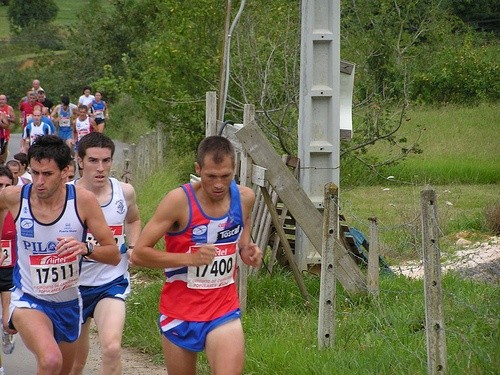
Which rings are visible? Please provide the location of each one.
[64,239,67,243]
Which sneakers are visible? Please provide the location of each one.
[2,329,15,354]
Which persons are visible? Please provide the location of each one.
[130,136,263,375]
[0,134,121,375]
[67,132,141,375]
[0,79,110,353]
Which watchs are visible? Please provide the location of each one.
[83,241,93,256]
